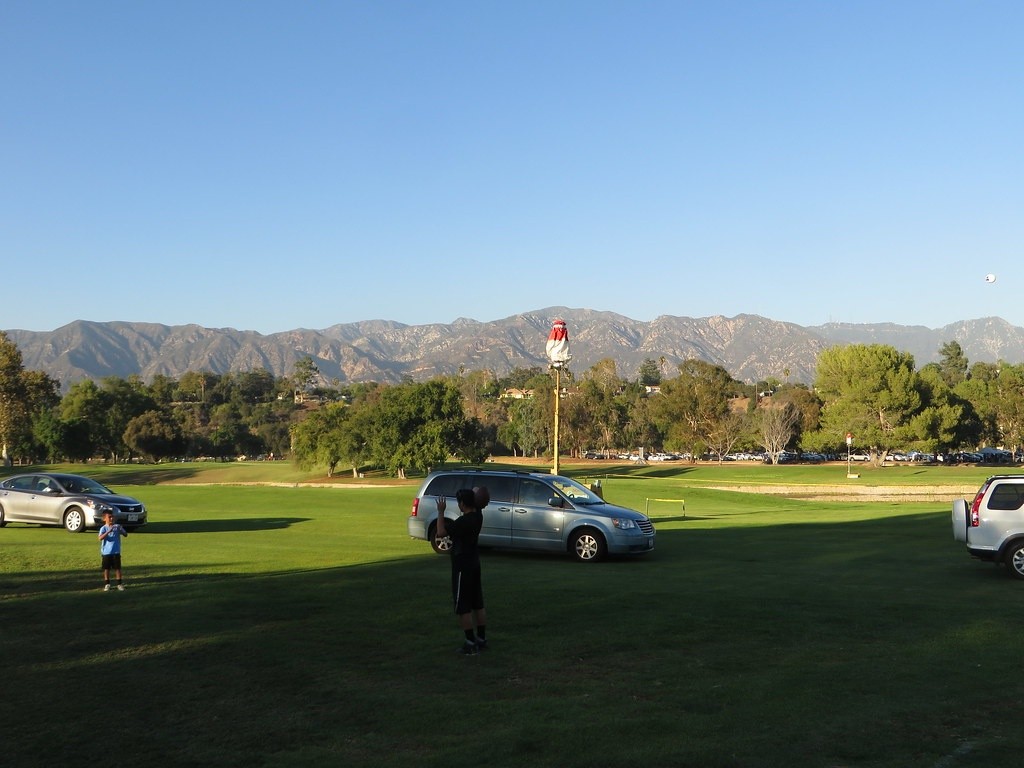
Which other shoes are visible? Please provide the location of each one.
[103,584,111,591]
[117,584,124,591]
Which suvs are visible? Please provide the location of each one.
[408,468,657,563]
[952,474,1024,581]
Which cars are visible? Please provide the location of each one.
[133,452,286,463]
[0,473,148,534]
[578,447,1024,464]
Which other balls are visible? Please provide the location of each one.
[472,485,490,508]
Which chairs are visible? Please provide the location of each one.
[13,482,23,489]
[37,483,47,490]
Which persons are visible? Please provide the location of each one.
[435,489,485,657]
[98,509,128,591]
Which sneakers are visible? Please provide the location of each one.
[476,636,488,650]
[456,639,478,656]
